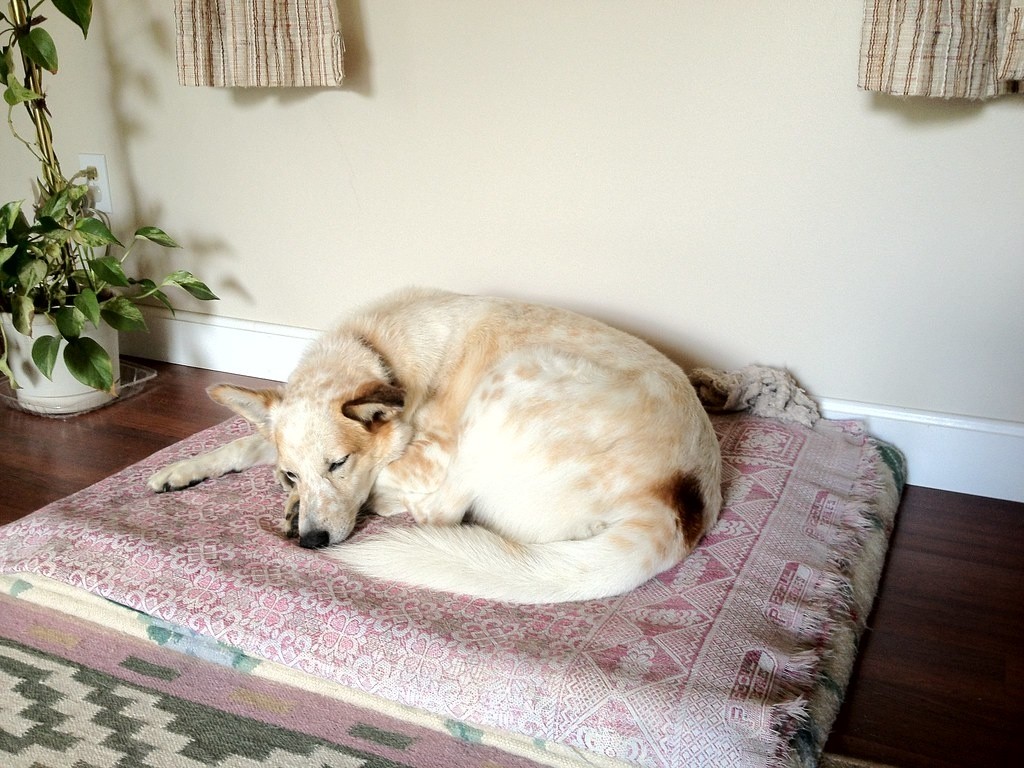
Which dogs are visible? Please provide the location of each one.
[145,287,722,603]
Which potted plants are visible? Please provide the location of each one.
[1,0,221,416]
